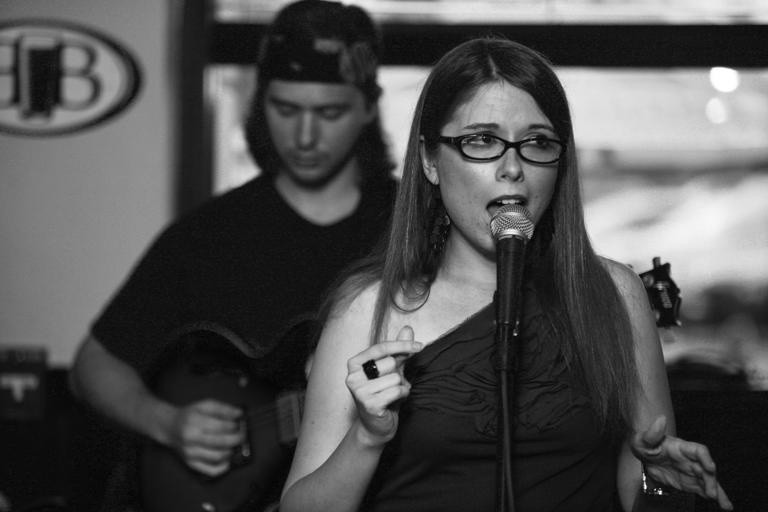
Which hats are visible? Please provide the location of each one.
[256,0,381,104]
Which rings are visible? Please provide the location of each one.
[358,359,381,382]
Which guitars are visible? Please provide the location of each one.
[139,255,683,510]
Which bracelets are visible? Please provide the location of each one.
[637,460,687,501]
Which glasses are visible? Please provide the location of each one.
[437,134,567,164]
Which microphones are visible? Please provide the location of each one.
[489,204,535,372]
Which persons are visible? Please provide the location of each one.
[277,36,739,511]
[68,0,413,510]
